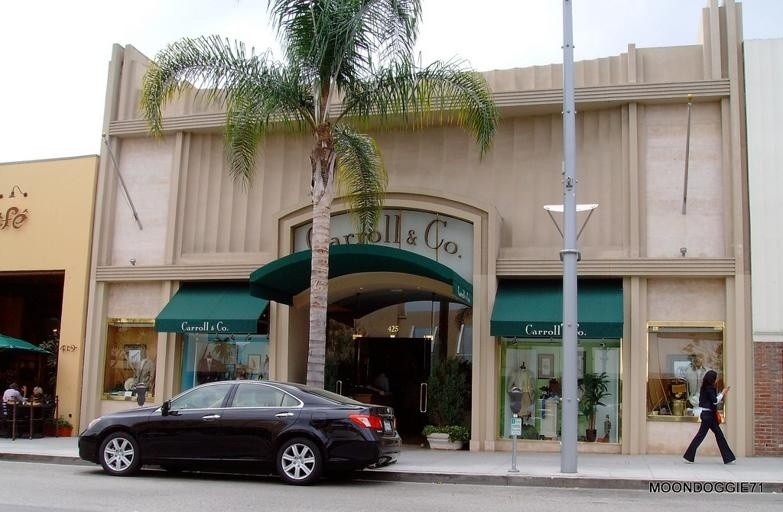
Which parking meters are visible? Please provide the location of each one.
[508,387,524,473]
[135,383,147,406]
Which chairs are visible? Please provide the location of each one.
[0,395,59,441]
[255,390,275,406]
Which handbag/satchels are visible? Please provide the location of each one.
[716,410,721,423]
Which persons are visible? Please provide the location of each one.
[682,354,716,409]
[2,382,46,418]
[133,351,156,385]
[682,370,737,465]
[508,362,534,416]
[199,352,222,384]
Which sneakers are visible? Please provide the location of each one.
[682,457,693,464]
[726,459,734,465]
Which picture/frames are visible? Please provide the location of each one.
[665,354,698,387]
[537,354,553,379]
[246,354,260,375]
[223,344,238,365]
[122,343,146,370]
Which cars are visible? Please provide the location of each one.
[77,380,402,486]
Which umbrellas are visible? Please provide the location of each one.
[0,333,56,356]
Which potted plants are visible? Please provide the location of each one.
[420,356,471,450]
[44,415,74,438]
[578,372,612,442]
